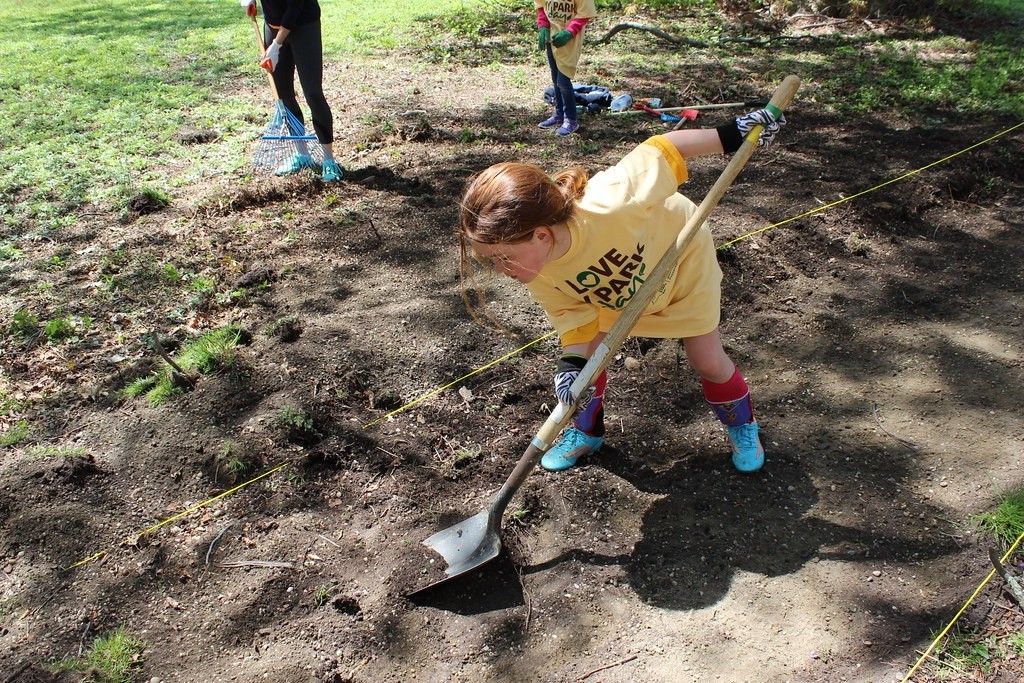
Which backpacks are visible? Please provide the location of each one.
[541,85,613,113]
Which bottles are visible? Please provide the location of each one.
[575,105,589,116]
[610,94,633,112]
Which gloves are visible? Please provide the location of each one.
[554,353,596,420]
[259,39,282,73]
[551,30,573,49]
[240,0,257,17]
[538,27,550,52]
[715,108,787,155]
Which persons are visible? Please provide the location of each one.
[458,109,788,474]
[533,0,595,136]
[239,0,343,183]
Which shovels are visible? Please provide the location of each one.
[404,76,801,594]
[673,110,699,130]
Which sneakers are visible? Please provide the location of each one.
[537,115,563,130]
[321,159,343,183]
[275,154,314,177]
[555,120,580,137]
[727,416,764,473]
[541,428,604,472]
